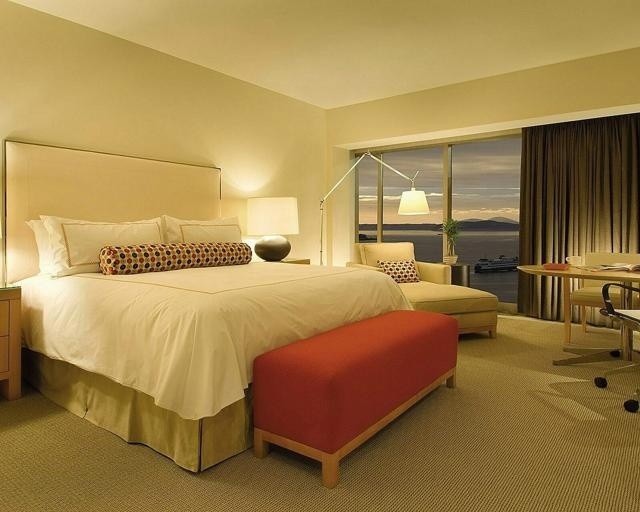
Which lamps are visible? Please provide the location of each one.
[318,151,430,267]
[246,195,300,261]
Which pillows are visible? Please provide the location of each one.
[98,243,254,275]
[361,240,415,265]
[39,215,164,275]
[29,219,50,277]
[375,261,418,283]
[165,214,244,246]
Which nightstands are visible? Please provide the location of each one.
[287,258,312,268]
[0,287,22,403]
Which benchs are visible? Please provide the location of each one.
[570,252,640,334]
[249,309,460,489]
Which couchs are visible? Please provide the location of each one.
[344,243,498,340]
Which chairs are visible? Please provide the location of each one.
[591,280,639,413]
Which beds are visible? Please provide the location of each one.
[5,140,416,474]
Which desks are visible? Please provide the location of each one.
[516,264,640,350]
[452,262,471,288]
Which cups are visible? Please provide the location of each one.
[566,256,582,265]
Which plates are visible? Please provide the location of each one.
[543,262,571,270]
[571,265,584,268]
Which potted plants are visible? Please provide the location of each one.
[440,218,461,265]
